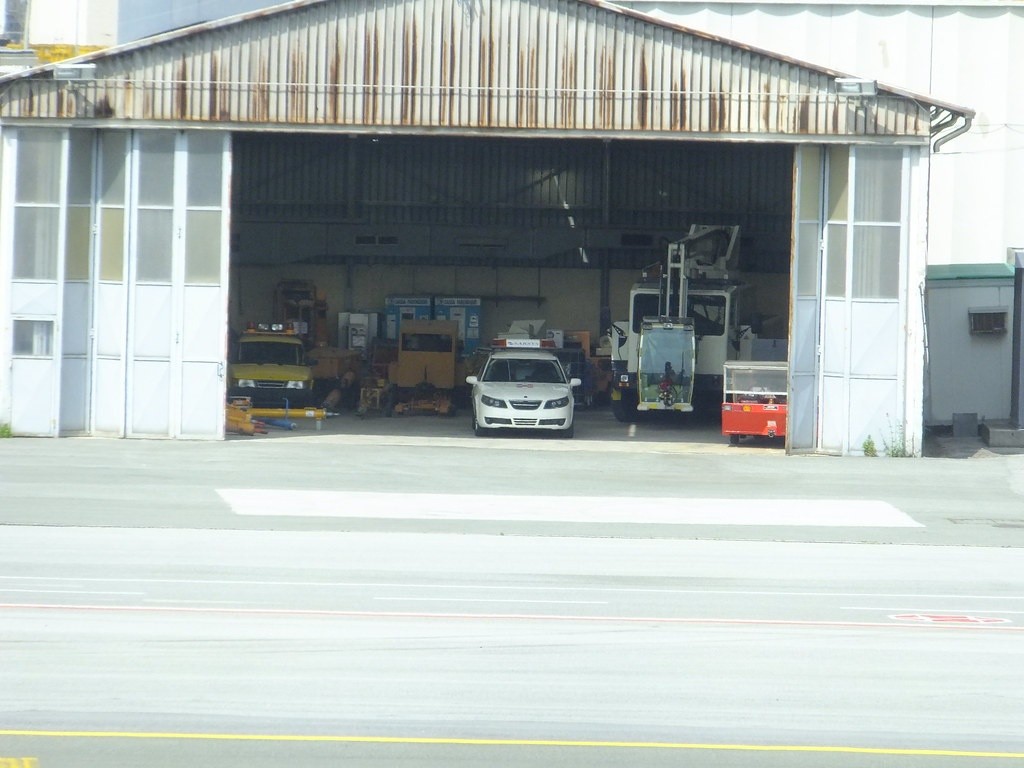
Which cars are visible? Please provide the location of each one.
[466,339,582,439]
[227,322,319,409]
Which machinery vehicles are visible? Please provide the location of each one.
[609,224,763,423]
